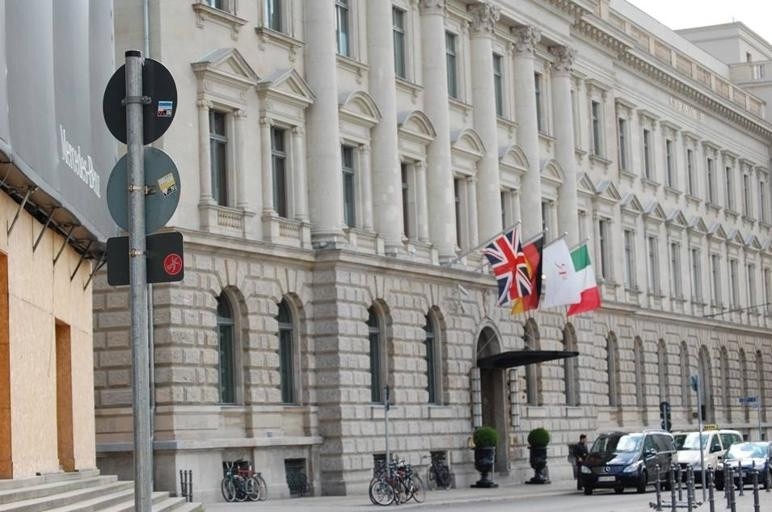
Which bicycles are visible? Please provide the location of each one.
[367,455,426,505]
[221,458,268,501]
[424,455,452,492]
[293,464,310,497]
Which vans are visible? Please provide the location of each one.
[577,429,678,495]
[672,424,741,490]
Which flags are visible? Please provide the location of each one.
[479,226,601,317]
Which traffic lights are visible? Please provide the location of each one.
[690,375,698,391]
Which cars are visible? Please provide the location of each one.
[715,441,772,490]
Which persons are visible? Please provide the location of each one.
[573,434,588,490]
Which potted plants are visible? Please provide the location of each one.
[471,428,499,489]
[525,428,551,484]
